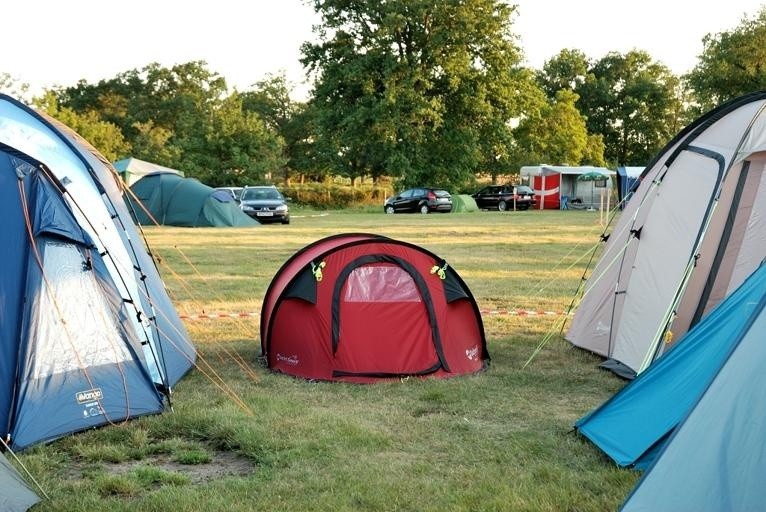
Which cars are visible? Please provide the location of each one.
[213,185,242,202]
[382,186,455,215]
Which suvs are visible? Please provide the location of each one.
[235,183,294,225]
[470,182,539,212]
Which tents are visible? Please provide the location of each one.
[0,438,49,512]
[111,156,262,228]
[615,166,647,211]
[0,92,198,453]
[560,93,765,381]
[260,234,491,383]
[574,260,765,511]
[450,195,480,214]
